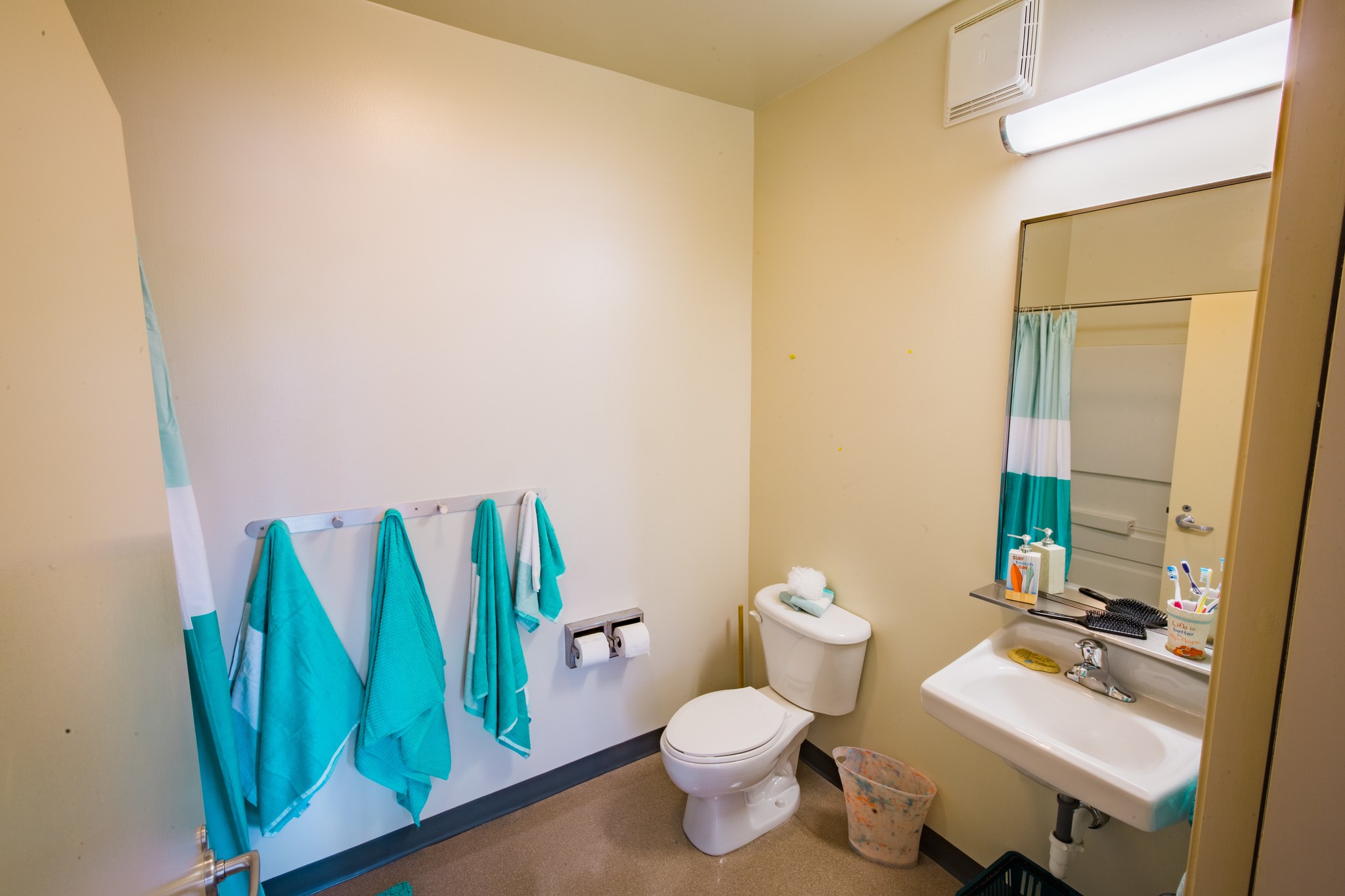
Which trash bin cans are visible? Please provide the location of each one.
[829,745,939,869]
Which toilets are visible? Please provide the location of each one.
[659,581,874,855]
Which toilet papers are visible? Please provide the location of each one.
[573,621,651,668]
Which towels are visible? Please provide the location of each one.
[215,491,567,840]
[778,586,834,618]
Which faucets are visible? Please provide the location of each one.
[1064,638,1136,703]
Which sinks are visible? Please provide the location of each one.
[919,631,1220,833]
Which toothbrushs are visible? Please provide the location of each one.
[1180,557,1226,598]
[1167,565,1229,613]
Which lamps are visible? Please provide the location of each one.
[1000,17,1292,159]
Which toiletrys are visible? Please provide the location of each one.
[1029,526,1066,596]
[1005,533,1042,605]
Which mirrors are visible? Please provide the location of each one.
[986,169,1274,652]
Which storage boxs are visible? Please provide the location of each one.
[955,849,1084,896]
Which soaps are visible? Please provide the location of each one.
[1008,648,1060,673]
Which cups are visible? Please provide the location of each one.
[1166,598,1214,662]
[1189,586,1223,646]
